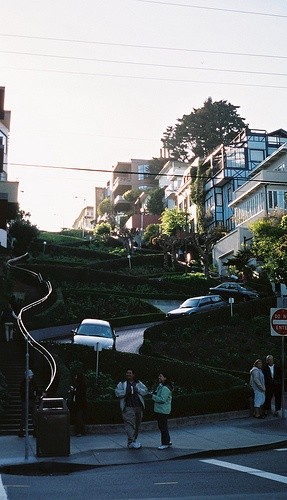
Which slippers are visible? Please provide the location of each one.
[253,414,265,419]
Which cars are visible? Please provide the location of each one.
[71,318,119,351]
[208,281,265,303]
[165,295,228,317]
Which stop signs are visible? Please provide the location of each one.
[271,309,287,336]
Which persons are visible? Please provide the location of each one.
[114,369,147,449]
[18,369,39,438]
[68,373,89,437]
[248,359,266,419]
[149,372,174,450]
[262,355,282,416]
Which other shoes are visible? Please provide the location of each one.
[127,442,141,449]
[262,413,269,416]
[158,442,172,450]
[273,414,280,417]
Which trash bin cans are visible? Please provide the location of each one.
[36,398,70,456]
